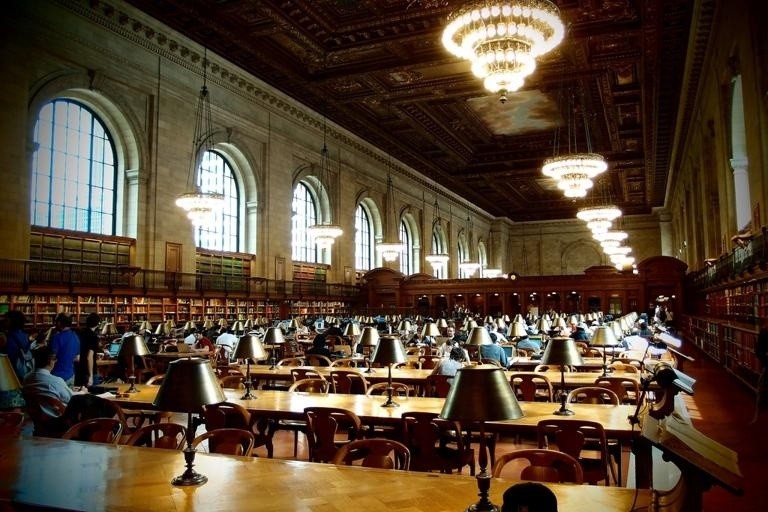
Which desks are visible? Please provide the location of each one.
[1,434,653,511]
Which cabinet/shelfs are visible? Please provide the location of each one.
[677,273,768,396]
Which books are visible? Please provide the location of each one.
[681,275,768,389]
[0,294,346,334]
[656,332,682,348]
[656,415,745,491]
[95,391,117,399]
[643,358,698,393]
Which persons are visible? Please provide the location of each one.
[121,320,653,388]
[2,311,109,387]
[750,326,767,423]
[23,346,88,426]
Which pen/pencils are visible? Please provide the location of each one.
[75,387,81,389]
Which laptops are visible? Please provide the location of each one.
[106,341,119,356]
[529,336,541,348]
[502,347,513,360]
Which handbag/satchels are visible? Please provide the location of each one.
[88,386,118,394]
